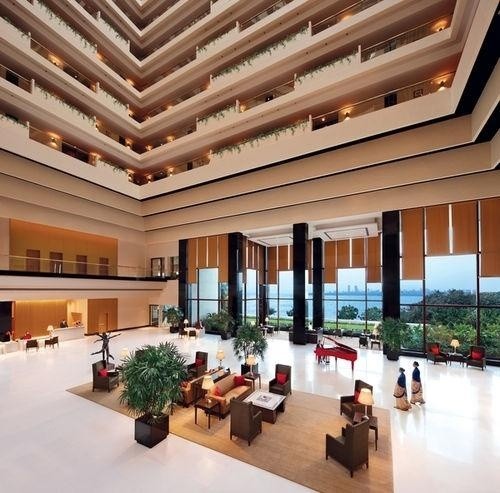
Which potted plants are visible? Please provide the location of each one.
[377,316,413,360]
[209,310,236,340]
[162,304,184,333]
[116,341,188,449]
[232,320,268,375]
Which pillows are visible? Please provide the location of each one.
[354,390,360,403]
[431,347,439,355]
[472,350,483,360]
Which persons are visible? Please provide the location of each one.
[317,339,326,362]
[392,367,412,411]
[409,360,425,405]
[23,330,31,339]
[60,319,69,327]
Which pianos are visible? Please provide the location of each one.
[314,336,357,380]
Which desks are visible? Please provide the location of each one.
[352,411,378,450]
[371,341,380,350]
[447,353,464,366]
[184,327,205,337]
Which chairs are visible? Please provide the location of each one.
[189,330,196,338]
[45,336,58,349]
[92,349,292,446]
[341,380,373,420]
[425,342,447,365]
[359,335,368,348]
[178,327,187,338]
[26,340,39,352]
[326,418,369,478]
[463,346,487,370]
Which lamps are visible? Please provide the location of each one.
[450,339,460,354]
[357,388,375,420]
[46,325,53,337]
[184,318,189,328]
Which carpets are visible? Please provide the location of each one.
[66,381,394,493]
